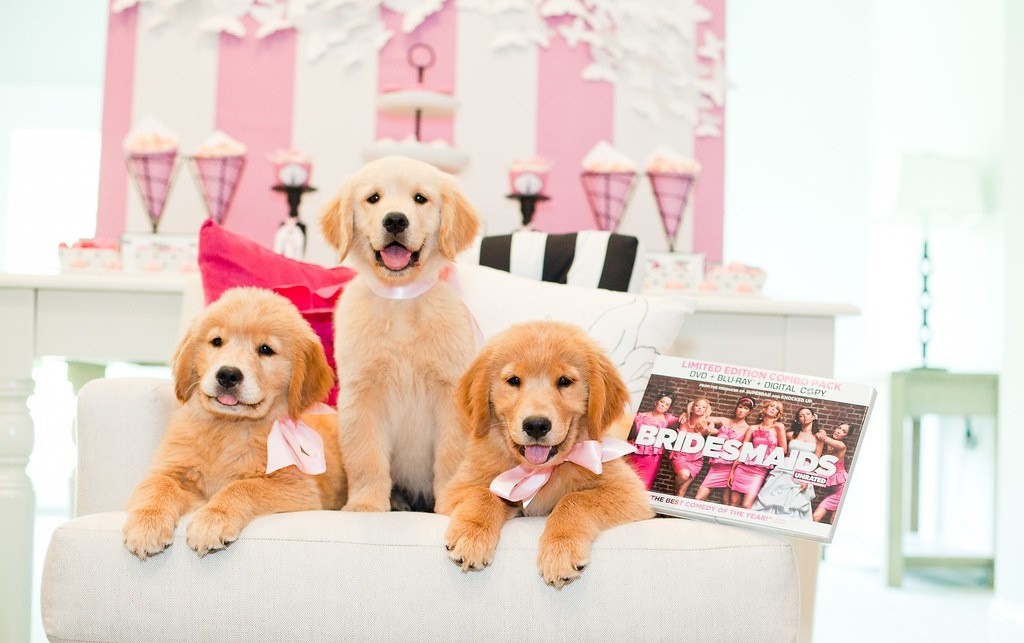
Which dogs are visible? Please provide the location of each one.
[319,155,492,514]
[120,286,347,562]
[440,320,657,590]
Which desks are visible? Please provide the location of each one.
[0,264,862,642]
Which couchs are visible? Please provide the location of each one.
[37,264,823,643]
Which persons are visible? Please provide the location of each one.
[626,391,855,524]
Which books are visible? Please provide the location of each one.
[623,354,877,544]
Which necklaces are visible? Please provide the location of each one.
[760,422,763,429]
[731,419,745,428]
[689,423,695,428]
